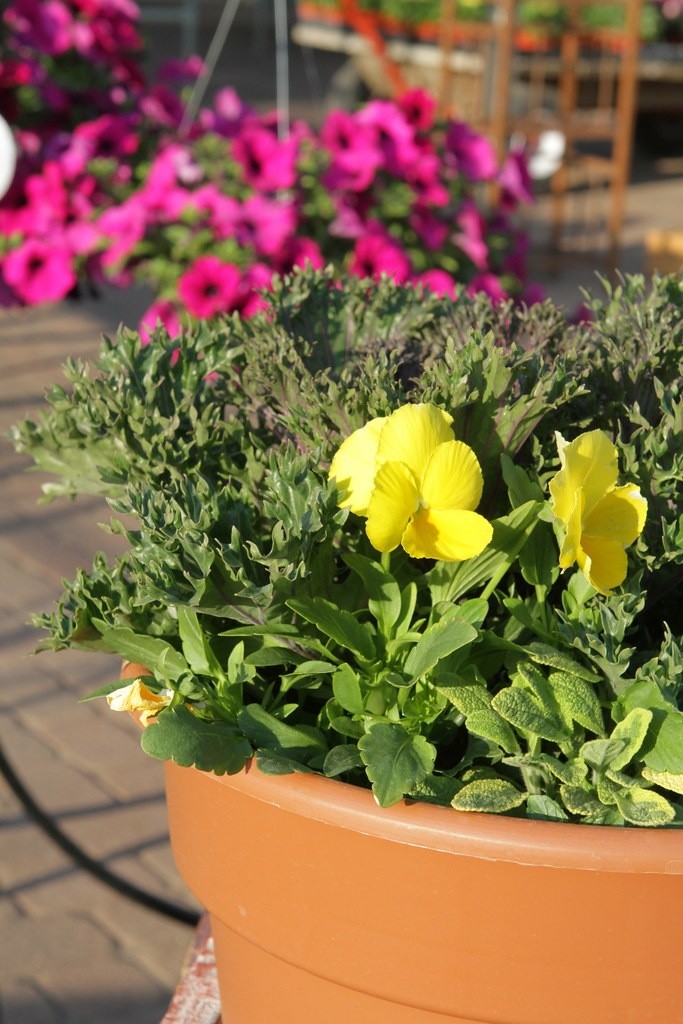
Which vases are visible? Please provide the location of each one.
[123,658,683,1024]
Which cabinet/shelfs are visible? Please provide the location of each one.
[440,1,640,295]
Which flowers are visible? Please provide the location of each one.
[2,260,683,831]
